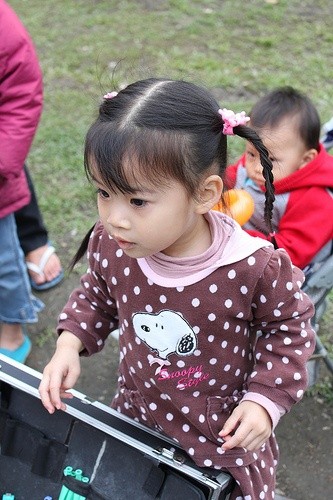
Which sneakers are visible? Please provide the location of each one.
[0,335,33,364]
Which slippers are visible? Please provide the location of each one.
[26,240,64,290]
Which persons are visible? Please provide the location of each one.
[12,162,66,293]
[37,75,318,500]
[213,83,333,401]
[0,0,46,366]
